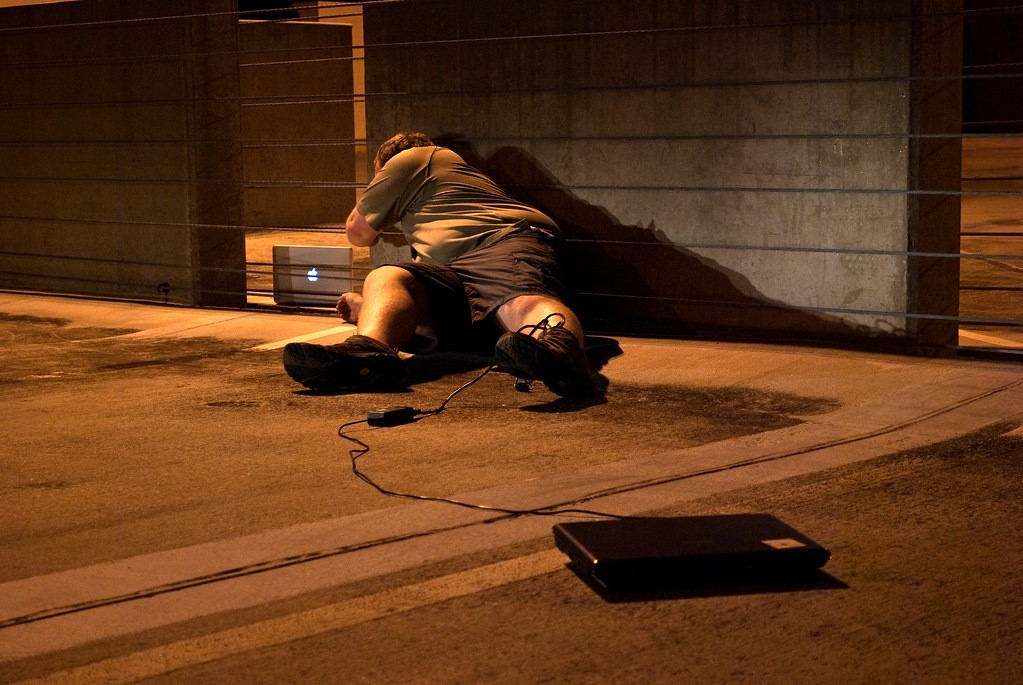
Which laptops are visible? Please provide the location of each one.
[552,513,831,593]
[273,245,353,307]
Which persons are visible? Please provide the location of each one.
[283,134,594,406]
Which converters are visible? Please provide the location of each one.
[367,406,414,426]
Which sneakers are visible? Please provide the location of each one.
[282,335,407,392]
[496,327,586,396]
